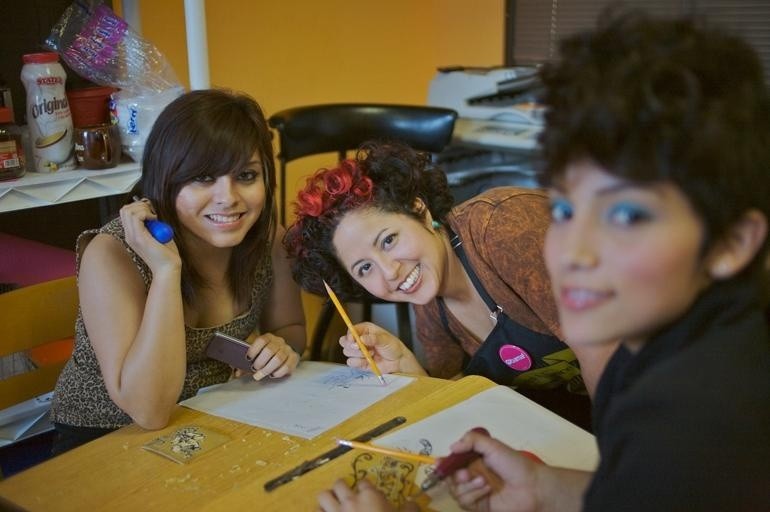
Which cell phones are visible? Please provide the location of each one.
[205,329,256,377]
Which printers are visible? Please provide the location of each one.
[425,64,553,153]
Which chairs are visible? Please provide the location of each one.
[269,103,457,361]
[0,276,84,411]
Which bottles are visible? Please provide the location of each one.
[20,51,78,173]
[0,106,29,182]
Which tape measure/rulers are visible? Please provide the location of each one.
[264,416,407,493]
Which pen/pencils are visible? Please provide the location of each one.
[337,427,492,491]
[323,280,387,385]
[131,194,175,244]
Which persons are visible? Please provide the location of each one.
[50,87,308,456]
[282,133,593,427]
[317,0,770,512]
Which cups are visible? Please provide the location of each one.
[74,121,122,171]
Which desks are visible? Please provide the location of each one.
[0,361,454,512]
